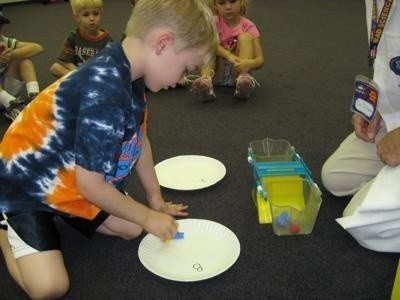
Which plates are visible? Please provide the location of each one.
[138,218,241,282]
[154,154,226,191]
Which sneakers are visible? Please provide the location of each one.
[4,98,26,126]
[24,92,41,106]
[233,73,262,99]
[189,74,217,102]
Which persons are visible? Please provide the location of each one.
[320,0,400,254]
[0,0,220,300]
[189,0,265,103]
[49,0,113,78]
[0,12,45,124]
